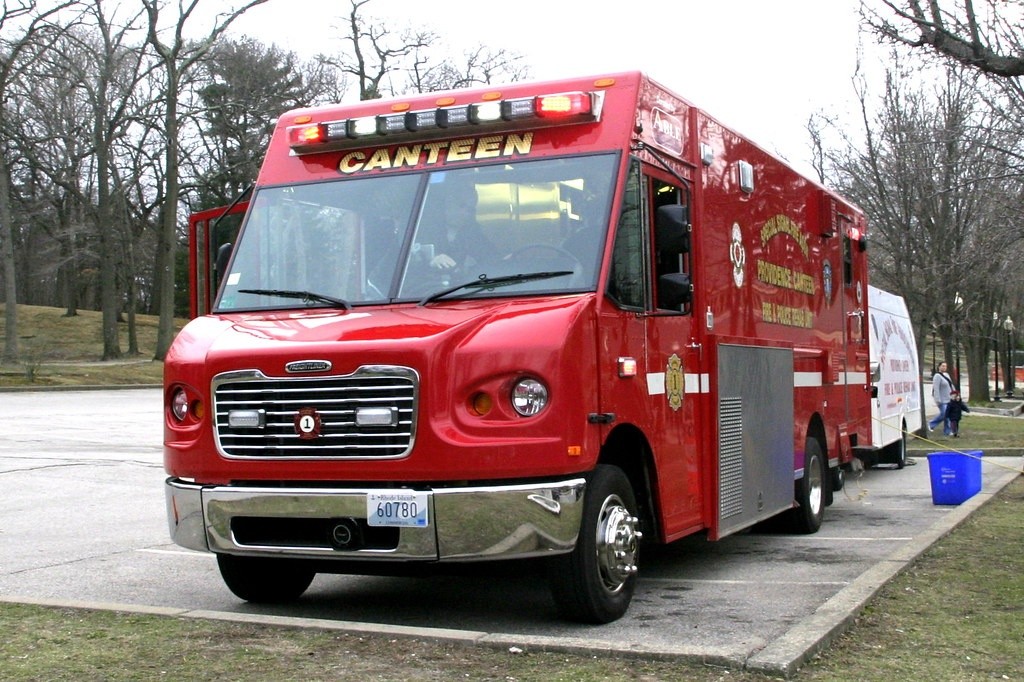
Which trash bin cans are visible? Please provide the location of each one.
[927,450,984,506]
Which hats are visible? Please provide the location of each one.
[950,390,961,402]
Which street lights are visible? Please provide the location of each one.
[1003,314,1015,398]
[991,311,1002,402]
[953,291,964,395]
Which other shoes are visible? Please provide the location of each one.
[928,421,934,432]
[949,431,953,435]
[954,433,960,438]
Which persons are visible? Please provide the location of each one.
[926,361,970,438]
[366,172,503,301]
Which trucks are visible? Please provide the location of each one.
[865,282,923,469]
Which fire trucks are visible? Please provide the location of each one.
[162,67,871,625]
